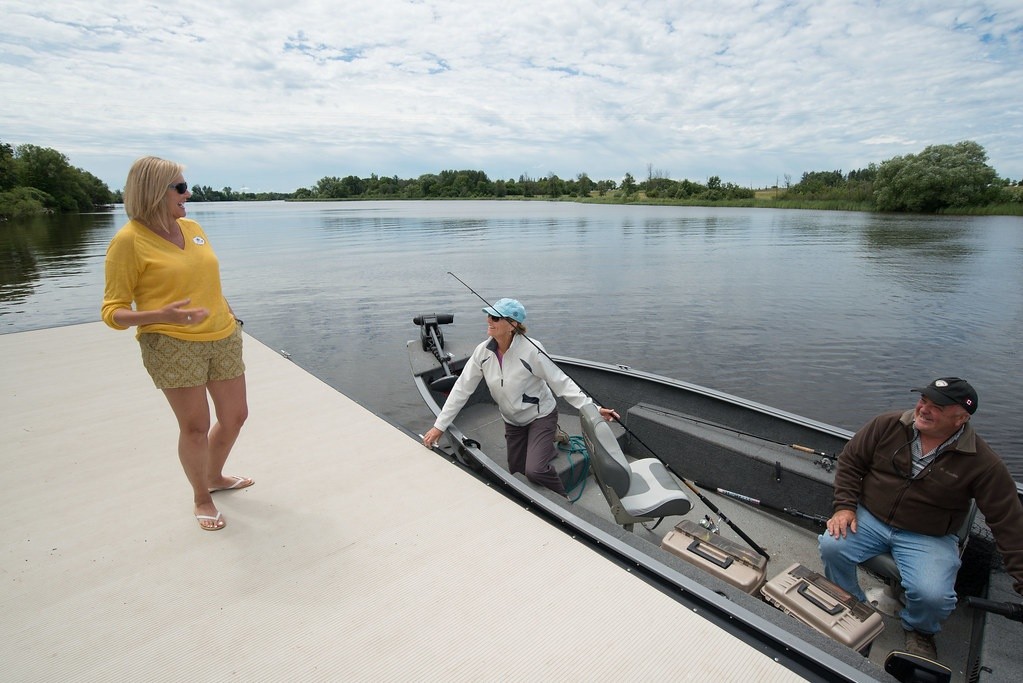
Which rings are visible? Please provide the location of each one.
[188,315,191,321]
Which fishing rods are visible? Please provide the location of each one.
[445,269,773,562]
[693,478,831,529]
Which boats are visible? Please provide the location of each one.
[410,312,1023,683]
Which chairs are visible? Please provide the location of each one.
[857,498,978,620]
[579,404,691,532]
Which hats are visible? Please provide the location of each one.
[481,298,526,324]
[910,377,978,415]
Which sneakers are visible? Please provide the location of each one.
[553,423,569,444]
[904,628,937,661]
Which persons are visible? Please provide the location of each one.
[101,155,256,531]
[423,298,620,498]
[817,377,1023,659]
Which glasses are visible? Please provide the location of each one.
[488,313,514,322]
[168,182,187,194]
[892,441,941,480]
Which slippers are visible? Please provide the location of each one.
[207,475,255,493]
[193,512,226,531]
